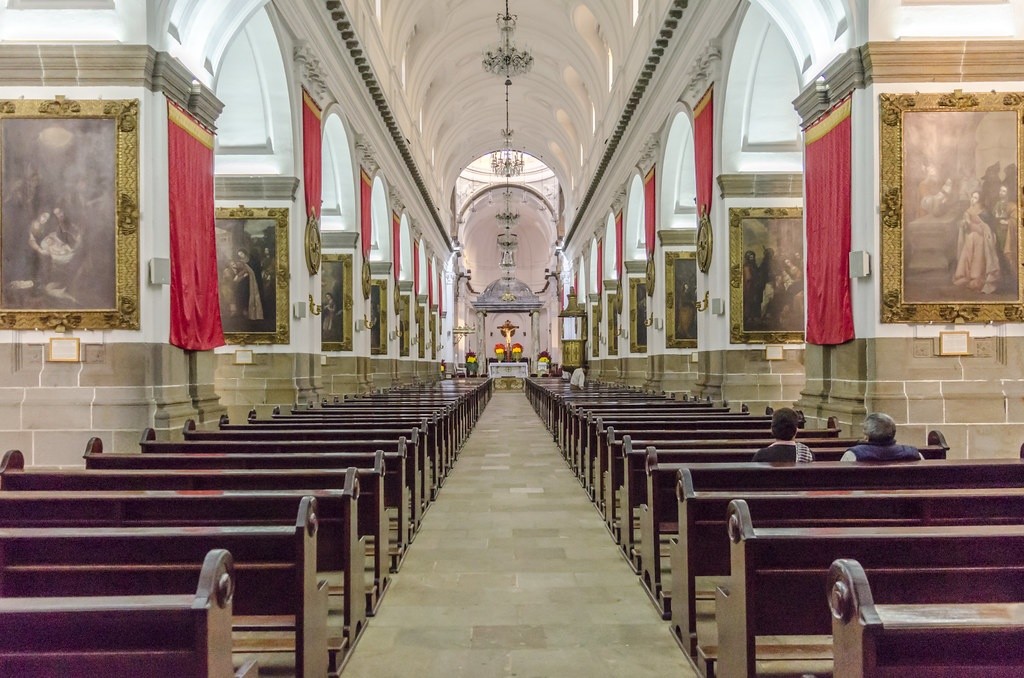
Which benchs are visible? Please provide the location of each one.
[2,370,1024,678]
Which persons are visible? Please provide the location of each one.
[840,411,925,460]
[570,365,590,392]
[750,406,816,462]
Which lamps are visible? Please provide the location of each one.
[480,1,533,303]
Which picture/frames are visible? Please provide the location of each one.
[0,96,142,333]
[727,204,805,344]
[416,306,425,358]
[320,253,352,352]
[608,293,618,355]
[429,315,436,361]
[592,304,599,356]
[371,279,388,355]
[664,250,698,349]
[399,295,410,355]
[878,90,1023,322]
[628,276,648,354]
[208,207,291,346]
